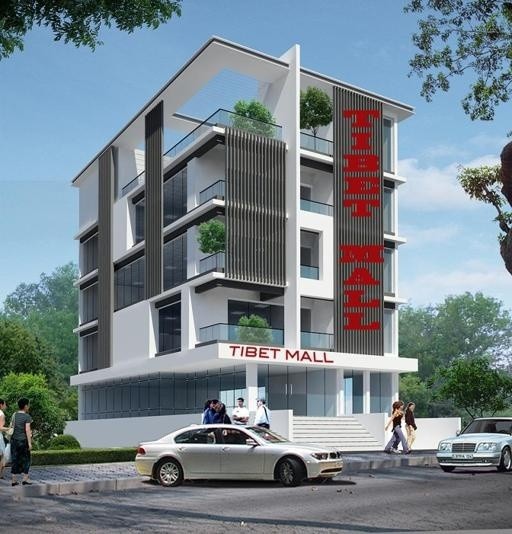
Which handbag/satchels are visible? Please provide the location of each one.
[5,444,11,462]
[5,428,14,440]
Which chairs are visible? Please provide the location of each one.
[192,431,247,443]
[484,424,495,431]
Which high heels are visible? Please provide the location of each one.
[12,480,32,486]
